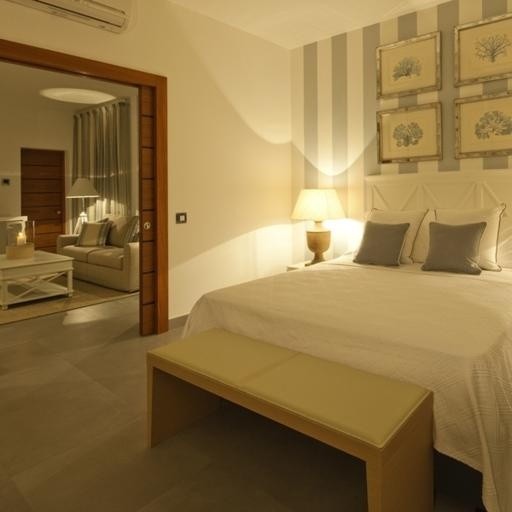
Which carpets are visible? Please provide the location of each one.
[0,275,140,326]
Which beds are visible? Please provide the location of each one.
[179,167,512,511]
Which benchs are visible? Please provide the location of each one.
[145,325,436,510]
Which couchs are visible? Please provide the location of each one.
[57,213,140,293]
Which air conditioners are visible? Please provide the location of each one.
[1,1,135,38]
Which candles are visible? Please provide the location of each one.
[17,232,27,246]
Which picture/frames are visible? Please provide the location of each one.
[373,29,443,102]
[451,90,512,160]
[451,11,511,88]
[375,100,444,166]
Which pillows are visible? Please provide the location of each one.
[101,213,139,249]
[350,203,507,276]
[74,221,112,247]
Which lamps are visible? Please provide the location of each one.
[63,177,102,235]
[291,186,347,266]
